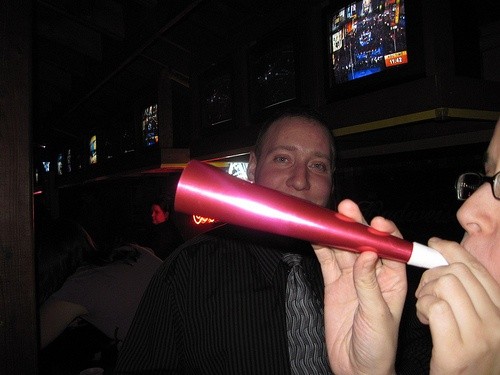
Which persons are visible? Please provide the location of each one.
[111,110,432,375]
[311,116,500,375]
[34,216,165,353]
[149,198,186,260]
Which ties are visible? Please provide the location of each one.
[280,252,333,375]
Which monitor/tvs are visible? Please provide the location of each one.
[140,103,160,148]
[88,135,97,165]
[327,0,409,88]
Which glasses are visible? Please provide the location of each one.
[456,171,500,202]
